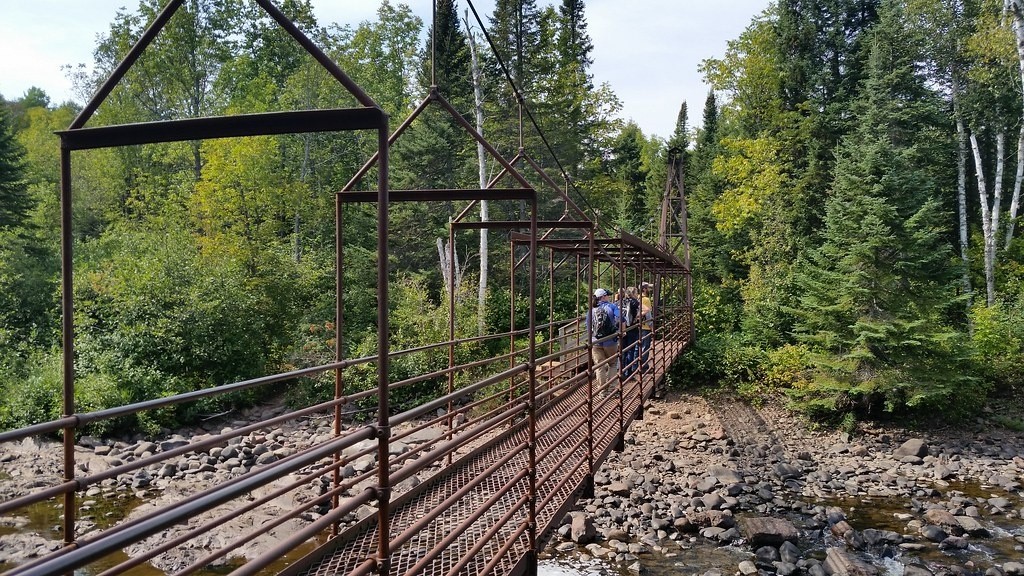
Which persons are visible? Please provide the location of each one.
[614,282,655,382]
[586,288,626,399]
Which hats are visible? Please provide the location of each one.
[592,289,607,299]
[604,289,613,295]
[639,282,653,290]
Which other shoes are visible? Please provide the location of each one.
[621,375,638,381]
[632,367,654,374]
[598,386,621,400]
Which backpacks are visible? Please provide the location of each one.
[617,301,632,326]
[592,302,619,339]
